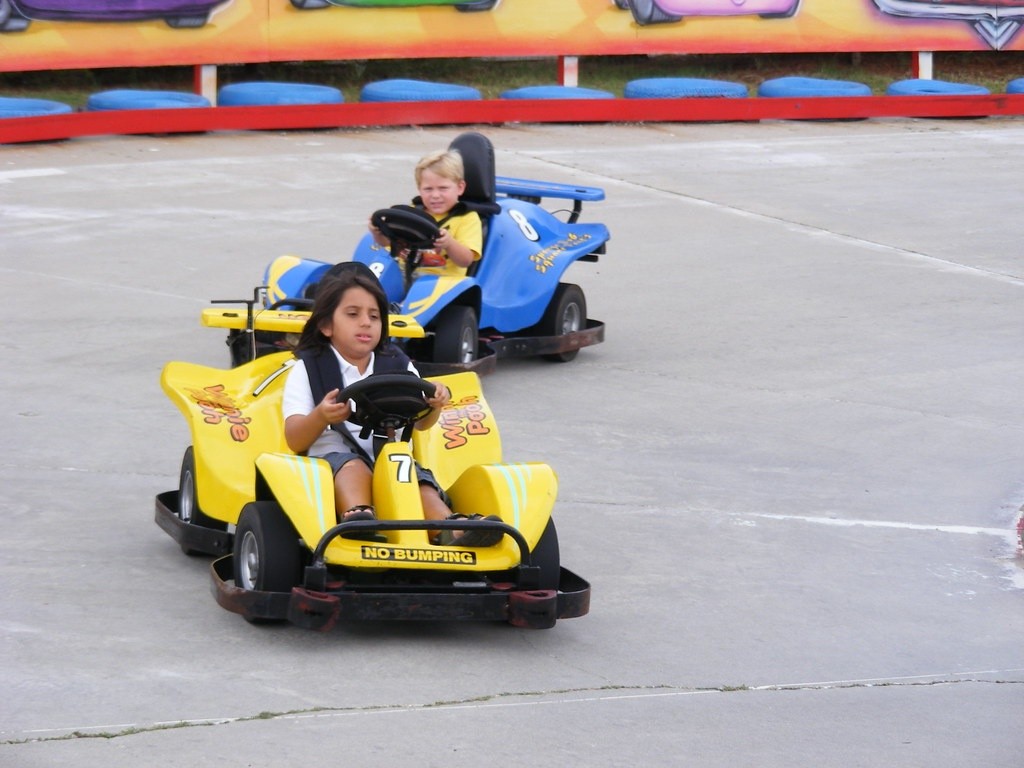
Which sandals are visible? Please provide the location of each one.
[431,513,505,547]
[341,504,377,522]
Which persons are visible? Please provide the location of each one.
[275,150,482,349]
[282,272,505,547]
